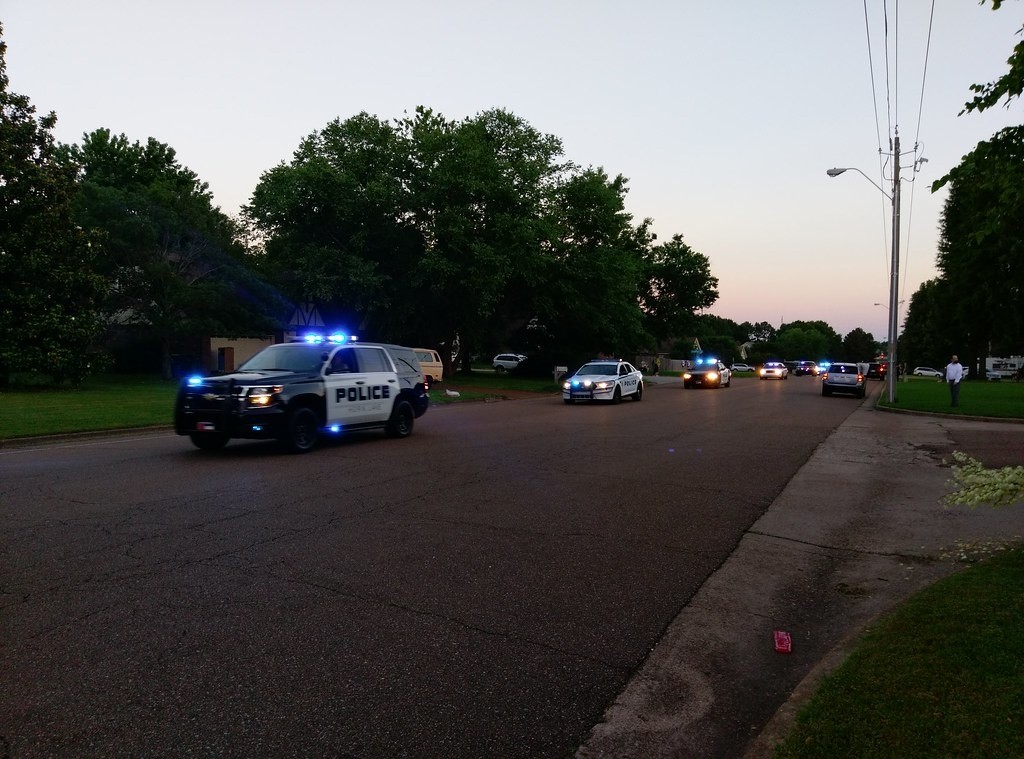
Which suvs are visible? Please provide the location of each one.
[492,352,528,375]
[821,362,867,400]
[173,335,431,455]
[863,362,885,381]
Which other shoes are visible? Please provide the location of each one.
[951,403,958,407]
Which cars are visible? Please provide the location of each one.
[783,353,888,376]
[730,363,756,373]
[684,359,731,389]
[562,359,644,405]
[913,367,944,378]
[795,360,818,377]
[961,355,1024,382]
[759,361,789,380]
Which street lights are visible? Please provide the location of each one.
[826,168,901,406]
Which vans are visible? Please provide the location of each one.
[411,347,443,389]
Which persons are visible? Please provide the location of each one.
[795,363,798,367]
[946,355,963,408]
